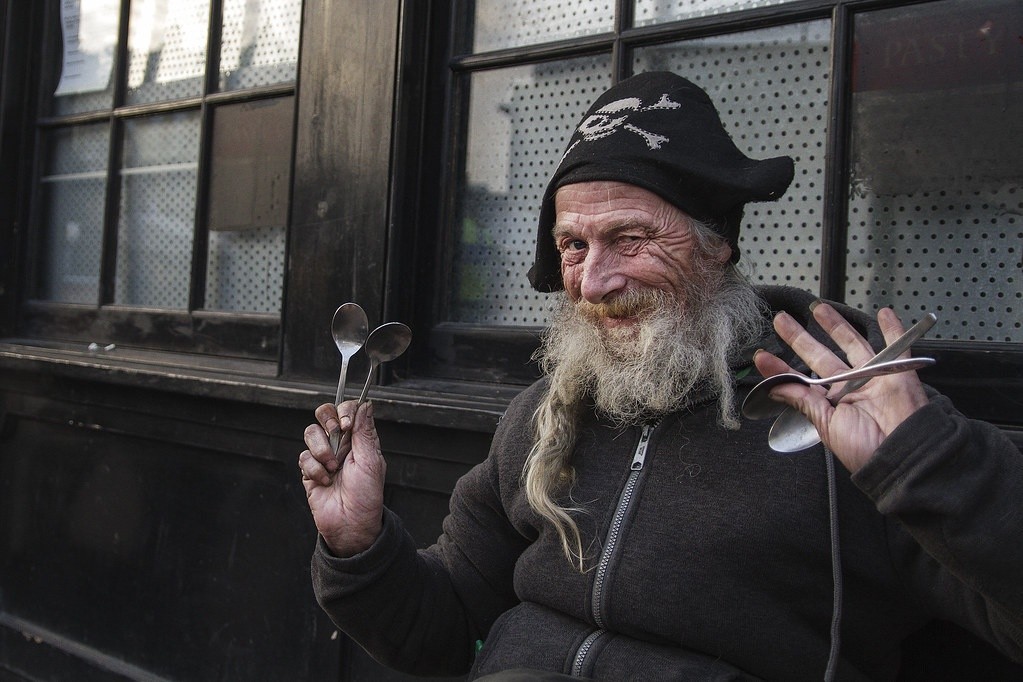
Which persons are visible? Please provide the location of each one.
[298,71,1023,682]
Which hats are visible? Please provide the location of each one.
[526,71,795,294]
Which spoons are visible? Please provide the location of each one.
[327,302,369,456]
[740,357,936,420]
[768,312,938,453]
[329,322,413,478]
